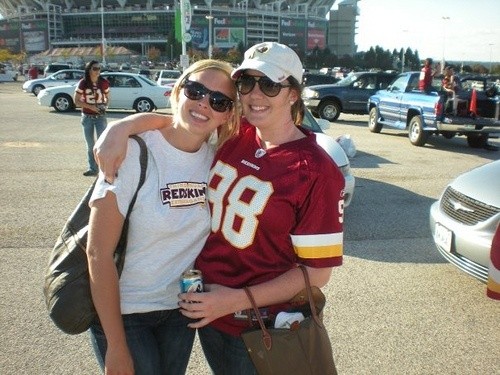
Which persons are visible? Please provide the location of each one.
[419,58,451,123]
[18,62,24,75]
[165,58,177,70]
[353,77,371,89]
[94,42,345,375]
[59,72,69,80]
[28,64,37,79]
[74,60,110,176]
[443,64,497,100]
[87,60,240,375]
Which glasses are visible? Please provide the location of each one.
[92,67,100,72]
[182,79,234,113]
[234,74,292,97]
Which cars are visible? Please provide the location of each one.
[301,71,341,87]
[20,63,45,80]
[22,69,87,97]
[157,69,182,88]
[427,160,500,294]
[0,64,18,82]
[37,71,173,114]
[460,74,500,117]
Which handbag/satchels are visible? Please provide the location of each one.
[239,266,339,375]
[43,134,147,336]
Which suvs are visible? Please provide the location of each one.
[45,64,75,79]
[301,71,403,122]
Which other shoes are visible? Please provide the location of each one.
[82,170,97,177]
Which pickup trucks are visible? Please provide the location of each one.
[368,69,500,147]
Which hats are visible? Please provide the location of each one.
[230,42,306,85]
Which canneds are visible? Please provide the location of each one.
[179,269,204,303]
